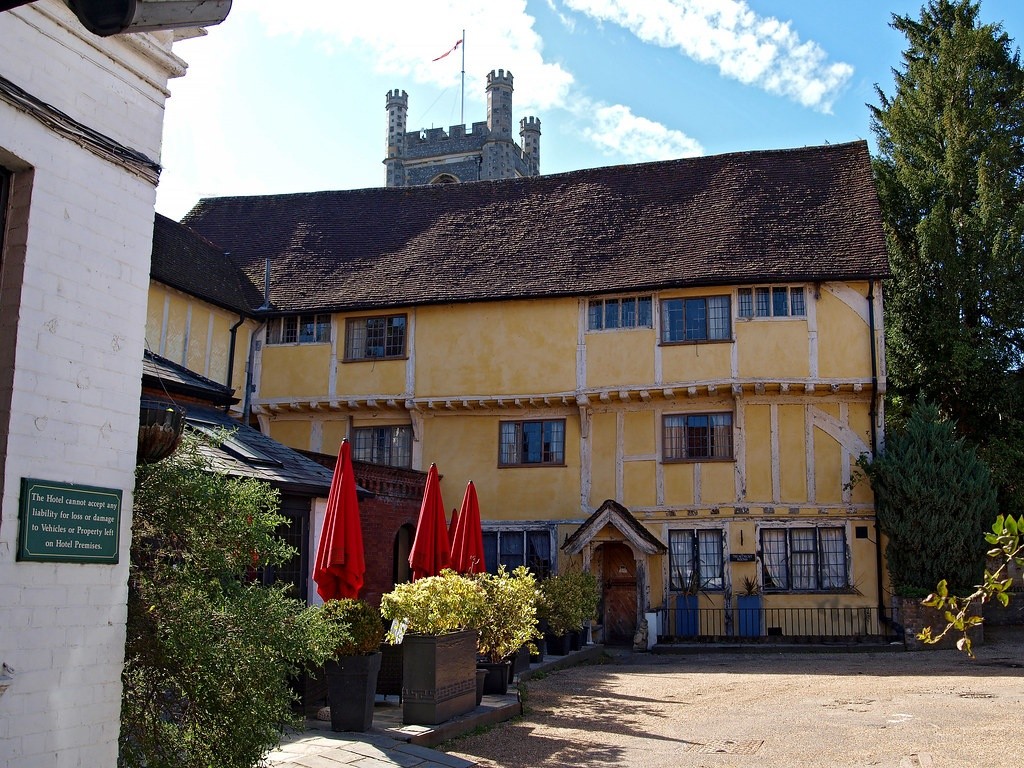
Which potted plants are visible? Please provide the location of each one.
[672,570,715,633]
[467,571,538,693]
[736,576,762,637]
[318,599,383,732]
[380,568,486,723]
[532,569,598,660]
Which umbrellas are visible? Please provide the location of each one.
[312,438,366,603]
[407,462,486,582]
